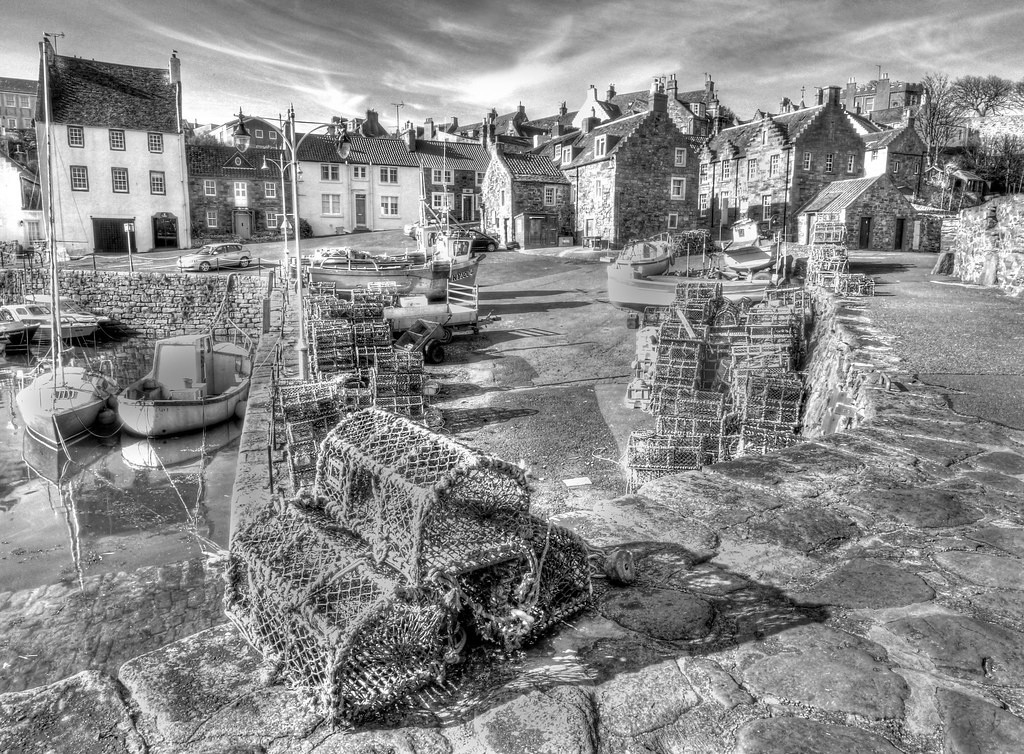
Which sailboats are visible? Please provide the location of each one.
[15,46,114,445]
[22,432,114,599]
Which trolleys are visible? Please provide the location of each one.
[384,279,480,343]
[394,315,452,364]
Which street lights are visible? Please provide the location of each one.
[230,103,349,383]
[257,155,299,287]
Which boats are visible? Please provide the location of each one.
[605,264,776,312]
[0,304,113,352]
[616,233,674,276]
[117,333,250,438]
[119,419,243,473]
[293,204,486,302]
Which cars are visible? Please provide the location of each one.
[181,243,253,272]
[448,229,499,251]
[404,220,421,240]
